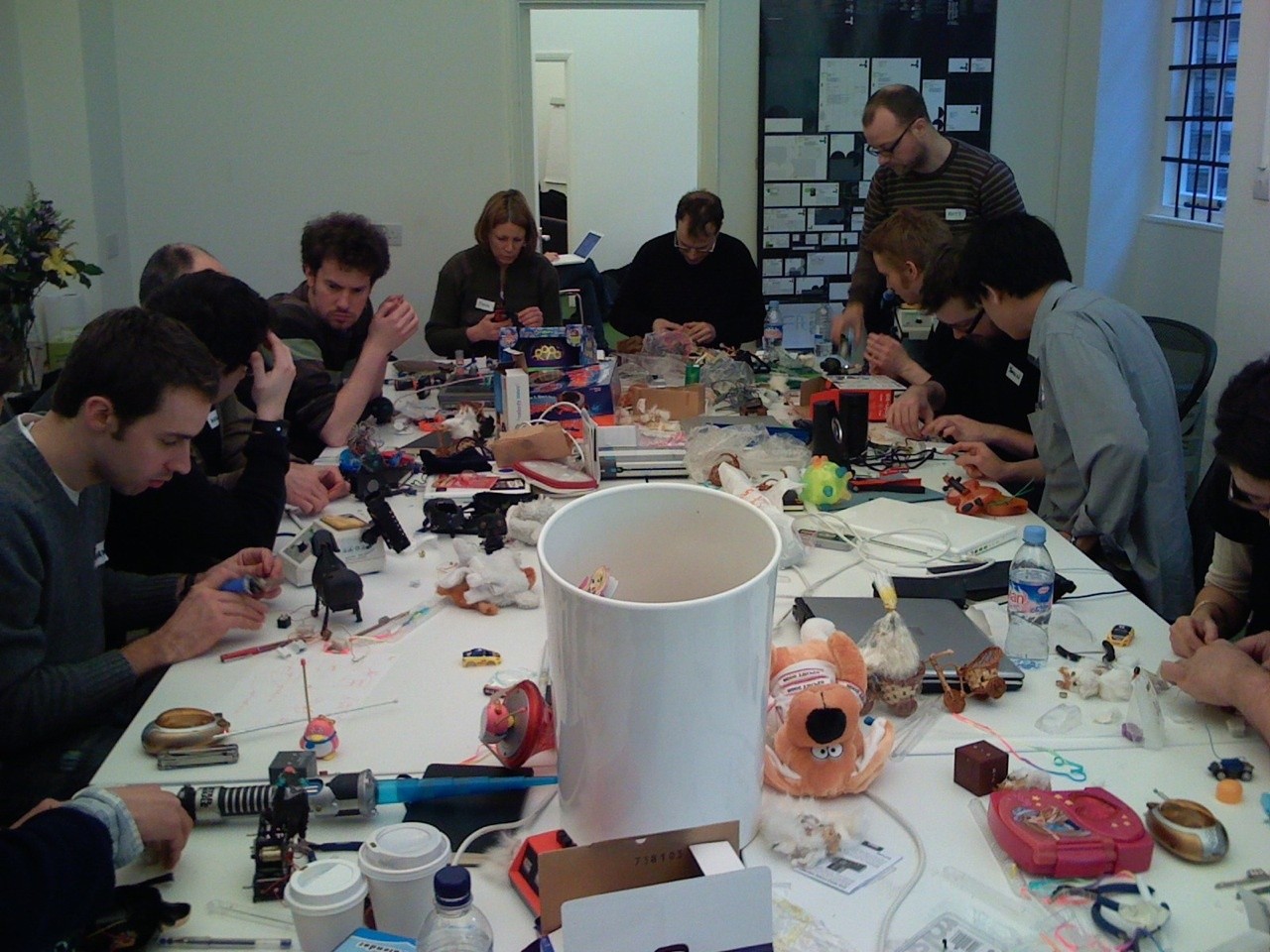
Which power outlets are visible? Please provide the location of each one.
[373,221,406,249]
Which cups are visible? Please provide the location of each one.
[358,822,452,937]
[283,857,369,951]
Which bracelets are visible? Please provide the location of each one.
[1190,600,1232,626]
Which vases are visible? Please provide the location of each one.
[0,301,35,375]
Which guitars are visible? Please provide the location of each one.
[942,475,999,514]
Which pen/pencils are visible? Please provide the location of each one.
[1215,875,1270,889]
[221,637,303,663]
[1237,885,1270,900]
[157,936,292,948]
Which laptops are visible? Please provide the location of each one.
[552,231,602,265]
[810,497,1016,565]
[793,596,1024,693]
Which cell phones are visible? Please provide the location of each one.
[494,309,507,324]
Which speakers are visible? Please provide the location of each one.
[839,393,868,456]
[812,401,852,466]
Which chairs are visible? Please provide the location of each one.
[1139,312,1220,423]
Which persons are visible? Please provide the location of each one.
[1165,356,1269,648]
[825,78,1029,364]
[137,241,355,518]
[1161,631,1269,745]
[604,189,765,355]
[422,187,564,362]
[920,212,1199,633]
[1,757,191,952]
[57,265,297,572]
[255,211,420,450]
[884,238,1045,461]
[1,305,284,809]
[854,203,969,385]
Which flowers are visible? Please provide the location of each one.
[0,177,106,392]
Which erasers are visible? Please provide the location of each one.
[1121,722,1144,741]
[1226,719,1246,737]
[1247,868,1266,878]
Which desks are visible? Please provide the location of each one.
[87,357,1270,952]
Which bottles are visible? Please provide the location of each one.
[814,307,833,357]
[1004,525,1055,671]
[763,300,784,368]
[414,864,494,951]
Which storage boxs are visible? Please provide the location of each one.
[534,819,776,952]
[628,384,707,423]
[486,420,573,469]
[47,325,83,370]
[493,323,624,440]
[794,371,909,425]
[491,343,534,432]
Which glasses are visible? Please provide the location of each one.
[674,232,716,252]
[945,308,986,334]
[1227,474,1270,511]
[867,116,923,157]
